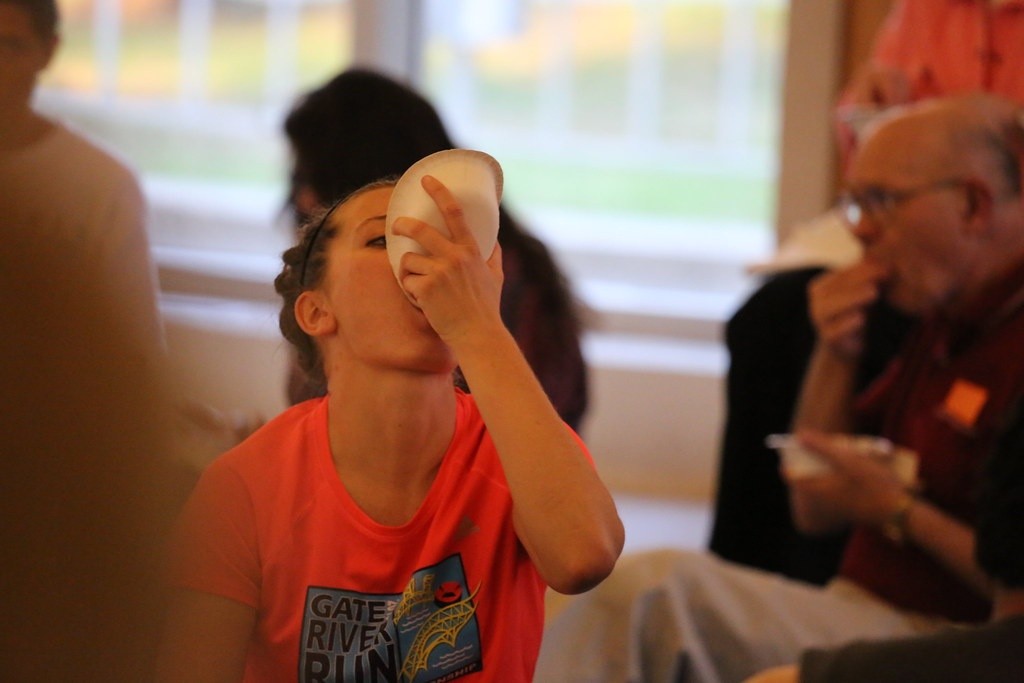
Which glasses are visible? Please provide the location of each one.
[835,177,964,235]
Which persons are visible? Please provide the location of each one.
[0,1,1024,683]
[150,175,625,683]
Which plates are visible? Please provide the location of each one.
[385,150,506,311]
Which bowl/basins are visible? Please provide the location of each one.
[767,432,894,478]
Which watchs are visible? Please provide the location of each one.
[882,494,912,540]
